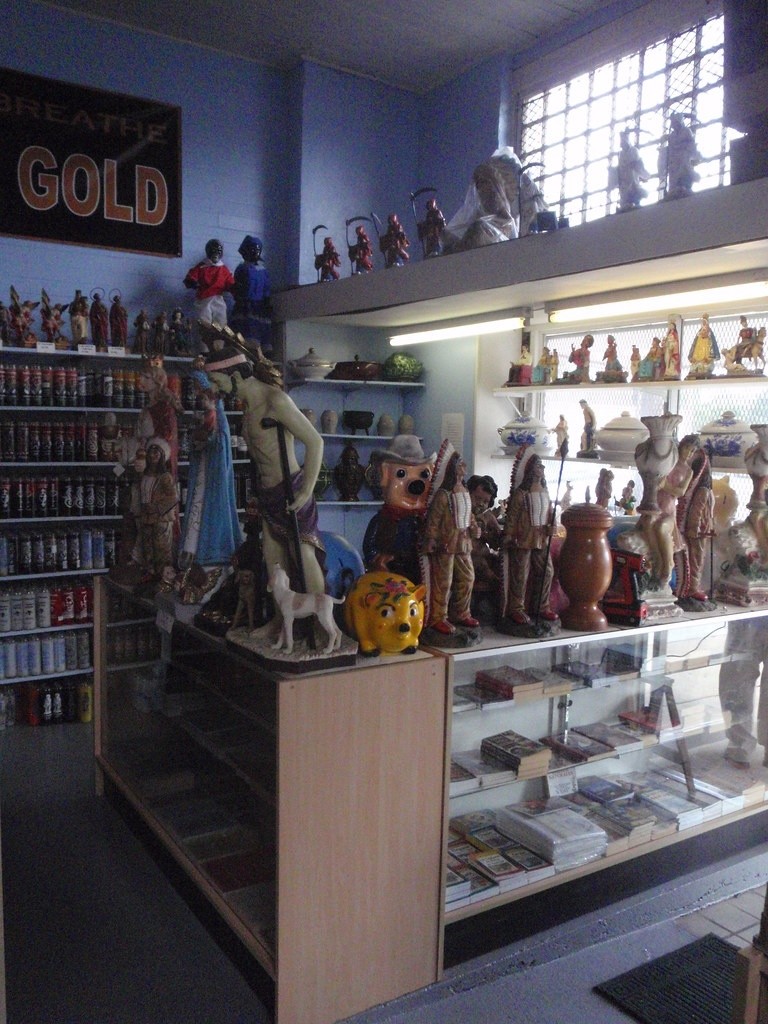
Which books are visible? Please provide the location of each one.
[445,660,768,912]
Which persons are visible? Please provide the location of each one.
[0,115,768,644]
[719,615,768,772]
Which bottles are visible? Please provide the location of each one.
[0,365,253,729]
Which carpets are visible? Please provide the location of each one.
[593,932,768,1024]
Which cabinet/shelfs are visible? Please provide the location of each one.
[0,348,768,1024]
